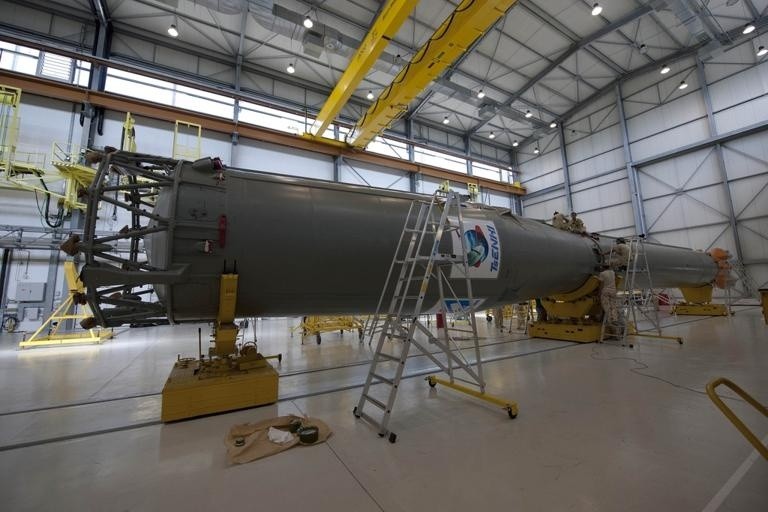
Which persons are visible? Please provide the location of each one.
[493,211,632,330]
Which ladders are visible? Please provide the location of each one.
[353,190,518,444]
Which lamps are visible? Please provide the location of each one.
[591,2,767,91]
[166,23,180,39]
[442,90,557,157]
[288,19,377,100]
